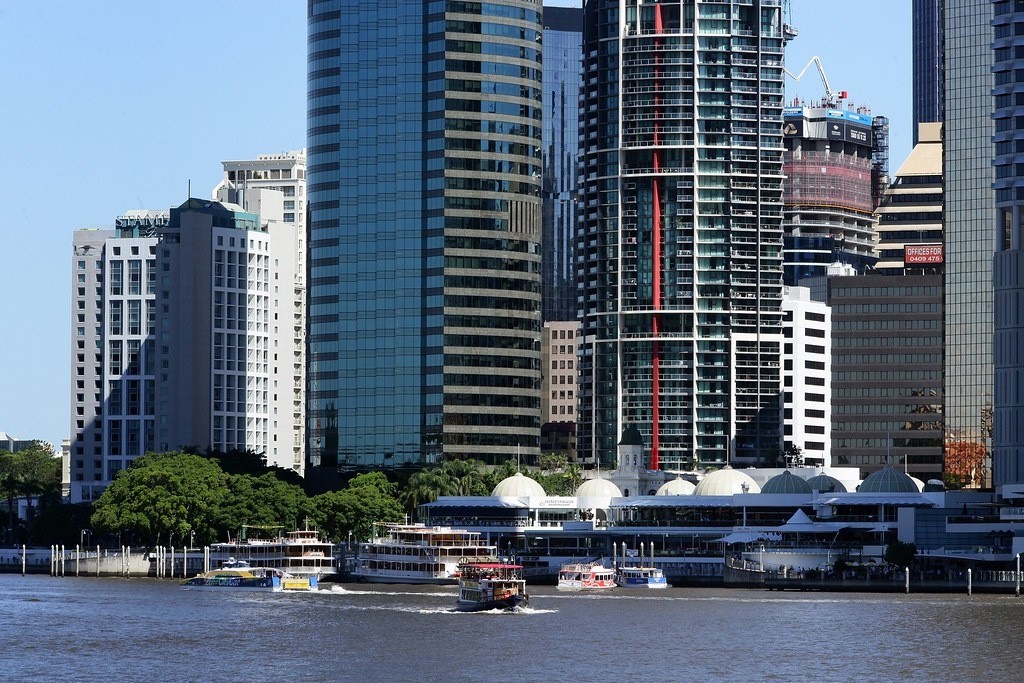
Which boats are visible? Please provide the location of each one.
[332,513,517,586]
[611,541,673,590]
[179,566,319,593]
[555,551,618,591]
[144,516,339,587]
[455,541,531,612]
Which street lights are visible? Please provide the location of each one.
[169,530,174,551]
[86,529,93,548]
[80,530,86,551]
[190,530,196,552]
